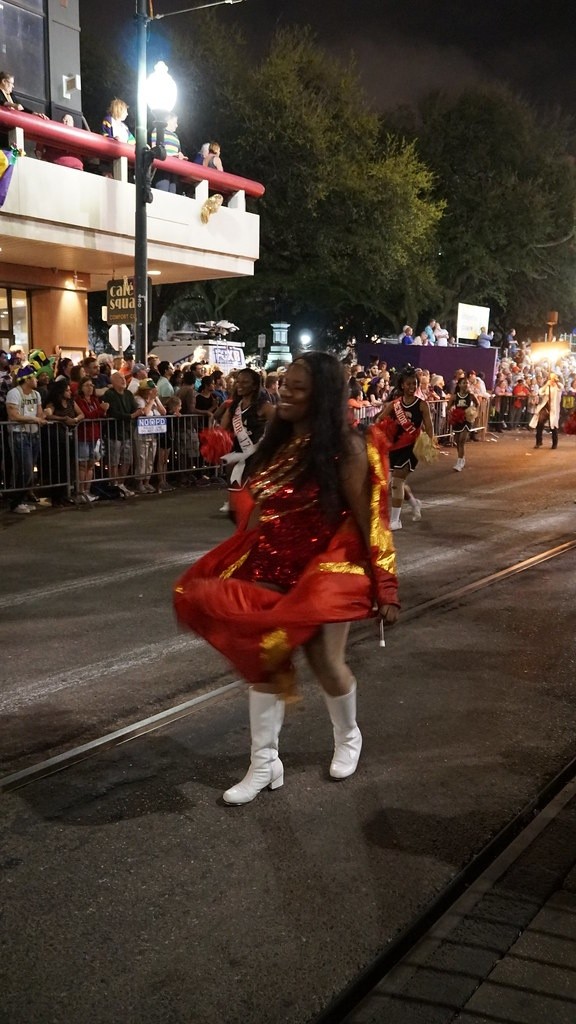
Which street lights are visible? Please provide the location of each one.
[133,0,243,382]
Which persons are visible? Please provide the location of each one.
[151,114,189,193]
[375,367,433,530]
[445,378,480,472]
[194,142,223,196]
[172,350,401,805]
[340,354,396,431]
[0,345,239,514]
[221,368,274,493]
[415,367,490,444]
[476,327,518,348]
[398,318,455,347]
[490,340,576,449]
[100,99,135,178]
[35,114,84,171]
[0,70,50,151]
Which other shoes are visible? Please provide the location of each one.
[25,504,36,510]
[158,483,176,492]
[135,485,151,493]
[219,501,230,511]
[115,483,135,497]
[81,491,99,502]
[14,504,30,513]
[146,484,157,494]
[552,444,557,448]
[453,465,465,471]
[533,442,542,449]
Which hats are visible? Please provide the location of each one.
[30,349,55,366]
[32,360,53,381]
[16,365,37,382]
[278,367,288,373]
[139,378,157,390]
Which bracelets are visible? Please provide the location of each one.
[74,418,78,422]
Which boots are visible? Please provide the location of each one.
[223,686,286,806]
[322,676,363,780]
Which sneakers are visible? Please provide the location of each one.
[390,520,402,531]
[412,499,421,522]
